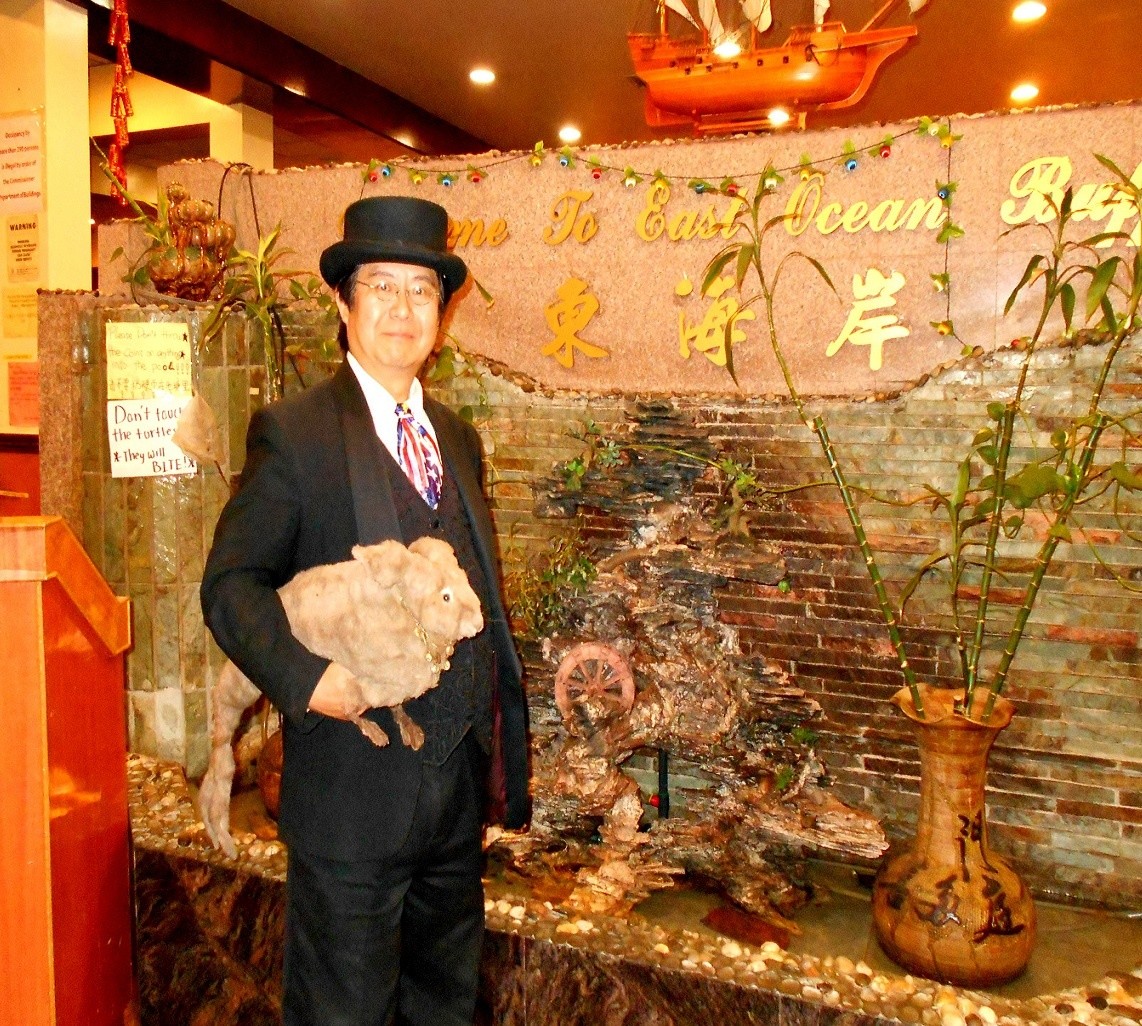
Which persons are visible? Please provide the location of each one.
[200,195,534,1026]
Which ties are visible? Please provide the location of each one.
[395,403,444,511]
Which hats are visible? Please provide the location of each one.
[319,196,466,303]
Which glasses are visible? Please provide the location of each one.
[355,280,446,305]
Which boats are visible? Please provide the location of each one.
[627,26,917,123]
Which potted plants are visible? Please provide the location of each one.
[687,123,1142,987]
